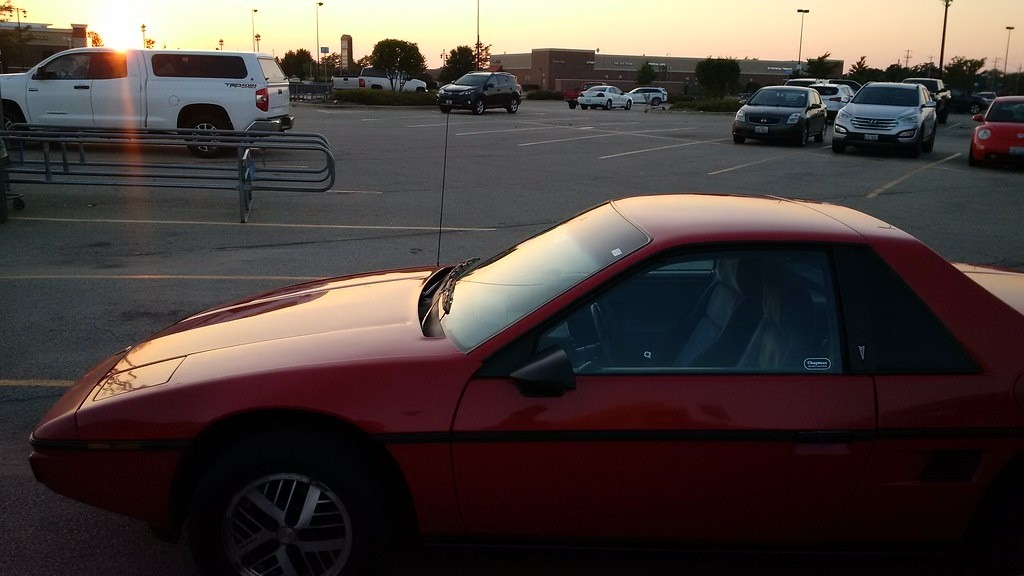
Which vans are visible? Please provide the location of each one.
[783,78,863,93]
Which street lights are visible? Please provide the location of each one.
[1000,26,1014,95]
[316,2,323,83]
[219,39,224,50]
[255,34,260,51]
[797,9,810,79]
[17,8,27,71]
[142,25,146,47]
[252,9,257,51]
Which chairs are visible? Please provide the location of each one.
[741,273,841,371]
[672,262,768,367]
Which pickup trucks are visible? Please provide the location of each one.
[902,78,951,125]
[578,86,634,111]
[0,46,293,157]
[330,67,427,93]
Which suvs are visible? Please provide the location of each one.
[437,71,523,115]
[625,87,668,105]
[808,83,855,121]
[832,81,937,157]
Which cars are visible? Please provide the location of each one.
[732,85,828,146]
[948,85,988,114]
[566,82,608,109]
[27,194,1024,576]
[969,95,1023,166]
[976,92,998,107]
